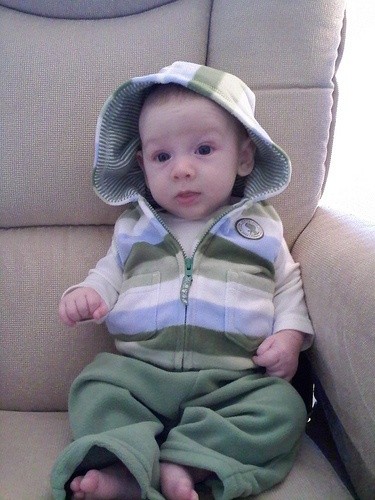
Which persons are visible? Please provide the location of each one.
[51,61,315,498]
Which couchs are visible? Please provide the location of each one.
[2,0,375,500]
